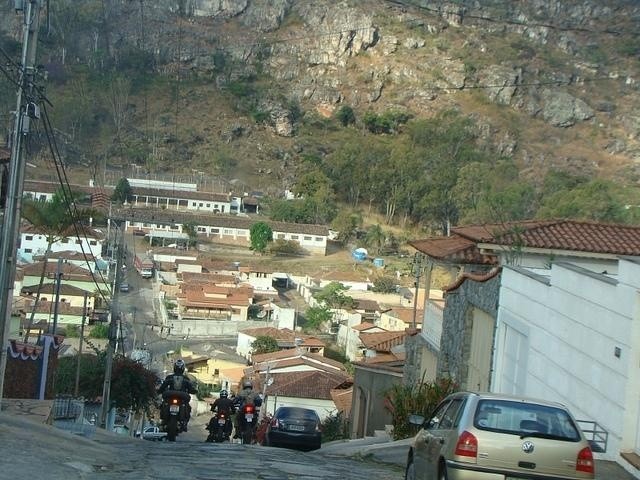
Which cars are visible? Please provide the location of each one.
[403,387,596,480]
[120,281,129,292]
[264,405,323,450]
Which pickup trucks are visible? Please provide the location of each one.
[135,424,169,442]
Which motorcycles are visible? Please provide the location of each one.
[153,384,200,442]
[231,402,262,445]
[208,402,237,442]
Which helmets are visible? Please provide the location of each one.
[174,359,185,373]
[220,390,228,398]
[242,381,253,390]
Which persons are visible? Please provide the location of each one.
[156,358,198,430]
[233,381,263,439]
[209,389,236,440]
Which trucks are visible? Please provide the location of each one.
[133,251,153,279]
